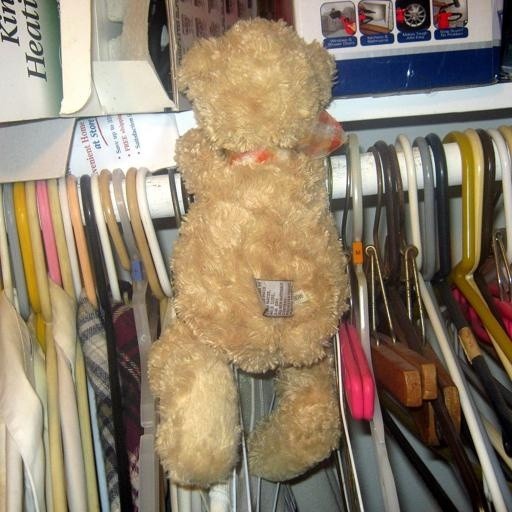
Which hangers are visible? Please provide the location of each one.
[0,125,512,512]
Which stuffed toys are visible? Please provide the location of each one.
[150,17,349,482]
